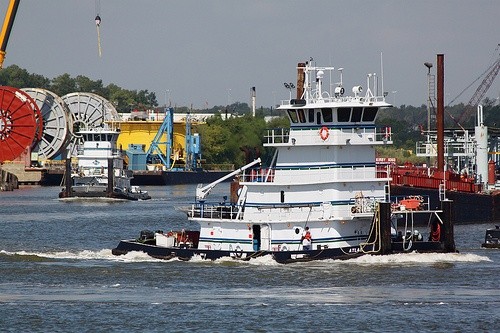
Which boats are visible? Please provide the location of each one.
[112,52,460,264]
[58,98,151,204]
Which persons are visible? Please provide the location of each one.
[301,227,311,250]
[241,146,271,183]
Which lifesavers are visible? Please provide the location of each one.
[390,203,401,208]
[319,127,330,141]
[381,126,390,139]
